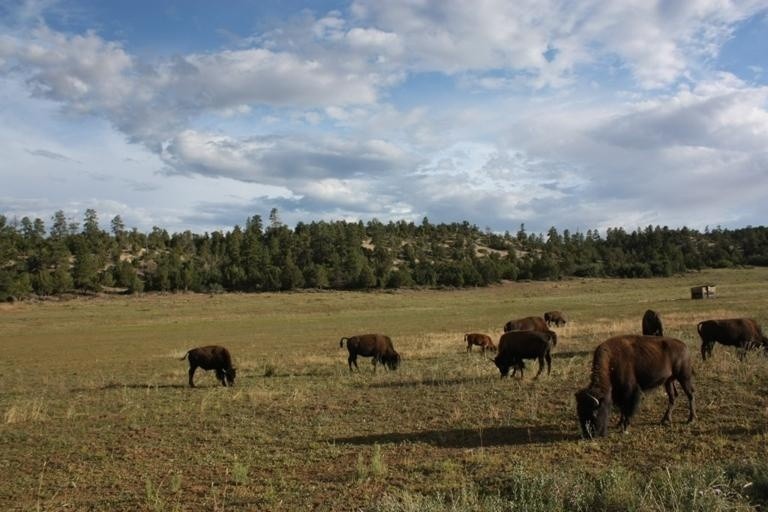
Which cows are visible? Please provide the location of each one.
[697,318,768,361]
[544,311,566,327]
[574,335,697,439]
[464,333,497,360]
[179,345,237,388]
[642,310,663,336]
[489,316,557,380]
[340,334,400,374]
